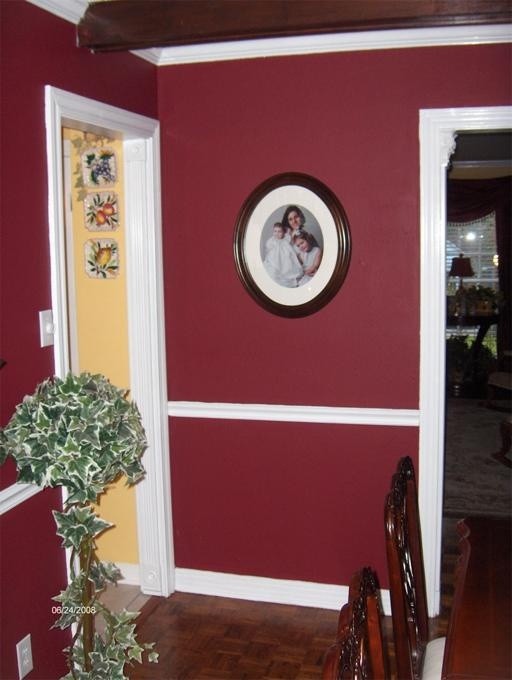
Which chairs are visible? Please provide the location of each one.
[485,350,512,413]
[385,455,446,680]
[321,568,391,680]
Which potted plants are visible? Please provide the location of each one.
[446,334,471,385]
[465,340,496,400]
[472,284,497,314]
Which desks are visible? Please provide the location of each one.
[441,517,512,679]
[491,413,512,469]
[446,315,498,344]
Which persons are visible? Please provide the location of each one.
[291,229,323,287]
[282,206,306,254]
[263,222,302,286]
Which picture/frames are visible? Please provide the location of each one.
[232,172,352,318]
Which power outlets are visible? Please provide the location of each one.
[15,632,34,680]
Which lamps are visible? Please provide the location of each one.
[449,253,475,296]
